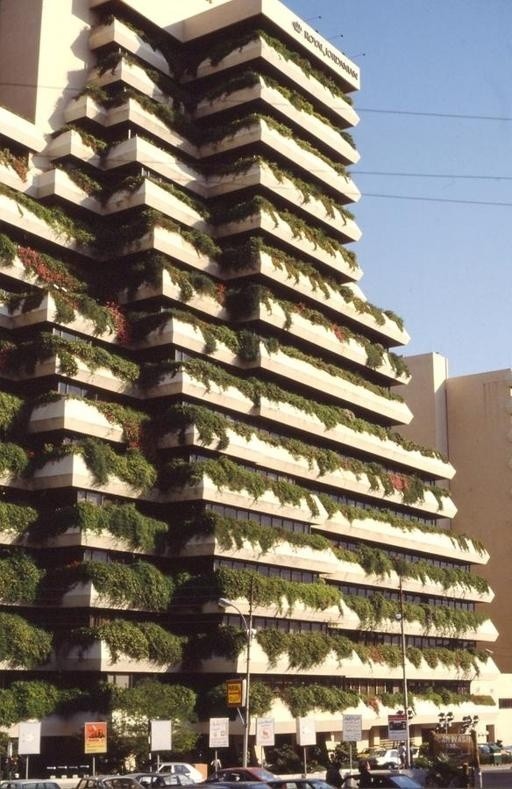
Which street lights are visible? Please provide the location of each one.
[439,711,453,733]
[215,598,252,767]
[463,714,479,742]
[398,710,414,766]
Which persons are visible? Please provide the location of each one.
[397,740,406,770]
[358,760,373,789]
[326,762,343,787]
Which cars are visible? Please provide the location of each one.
[356,741,511,771]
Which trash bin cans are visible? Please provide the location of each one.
[489,745,501,768]
